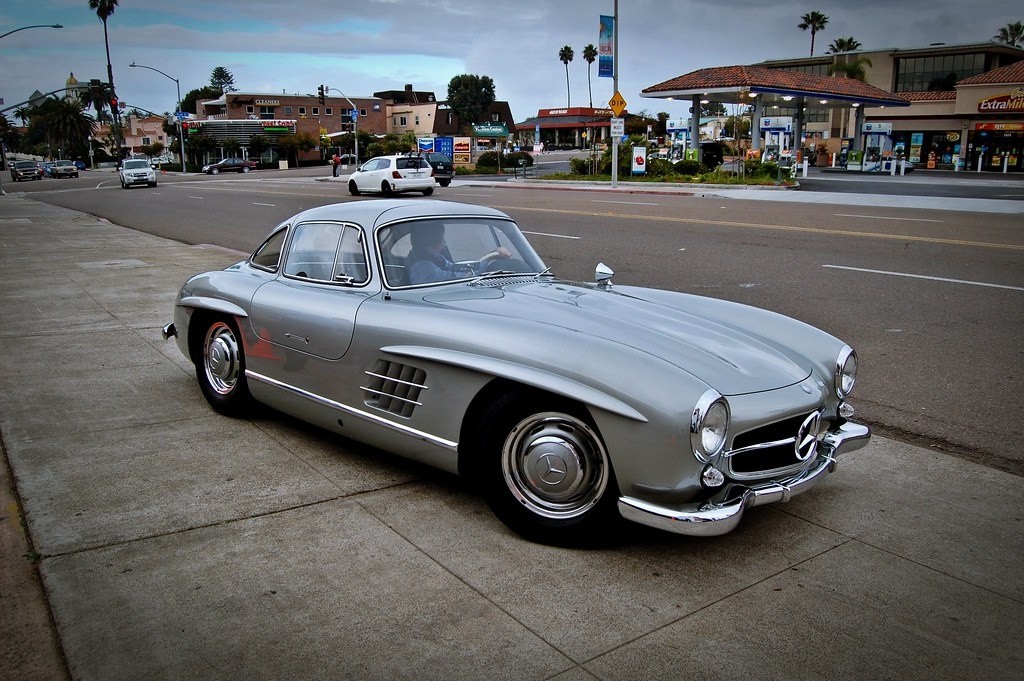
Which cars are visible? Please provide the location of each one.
[7,158,16,166]
[160,197,869,547]
[202,157,256,173]
[75,161,85,171]
[715,136,734,141]
[118,160,157,189]
[897,160,914,173]
[37,162,57,178]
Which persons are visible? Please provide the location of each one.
[331,154,341,178]
[405,222,511,284]
[539,140,551,154]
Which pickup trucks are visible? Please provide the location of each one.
[10,160,40,182]
[52,160,79,179]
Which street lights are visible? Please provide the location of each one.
[128,64,187,174]
[582,121,586,149]
[88,135,94,170]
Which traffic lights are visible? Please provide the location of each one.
[318,85,325,106]
[111,100,119,114]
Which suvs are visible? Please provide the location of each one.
[347,154,436,198]
[327,154,356,165]
[148,158,168,168]
[408,152,456,187]
[685,141,724,168]
[648,137,664,149]
[791,147,813,156]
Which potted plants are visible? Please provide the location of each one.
[815,143,830,167]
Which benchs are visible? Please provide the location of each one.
[258,249,404,289]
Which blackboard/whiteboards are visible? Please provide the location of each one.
[279,160,288,170]
